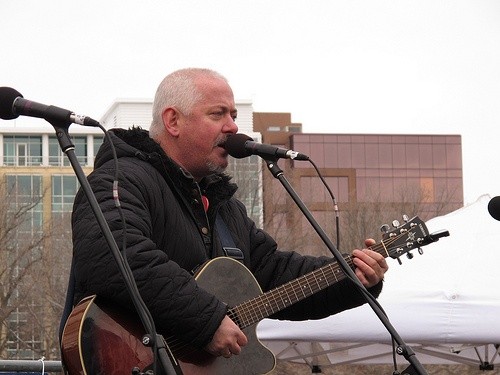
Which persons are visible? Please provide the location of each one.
[59,69,389,375]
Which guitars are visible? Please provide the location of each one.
[61,212,451,375]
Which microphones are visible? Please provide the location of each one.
[226,134,310,163]
[488,196,500,221]
[0,86,100,129]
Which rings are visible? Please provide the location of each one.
[223,351,232,358]
